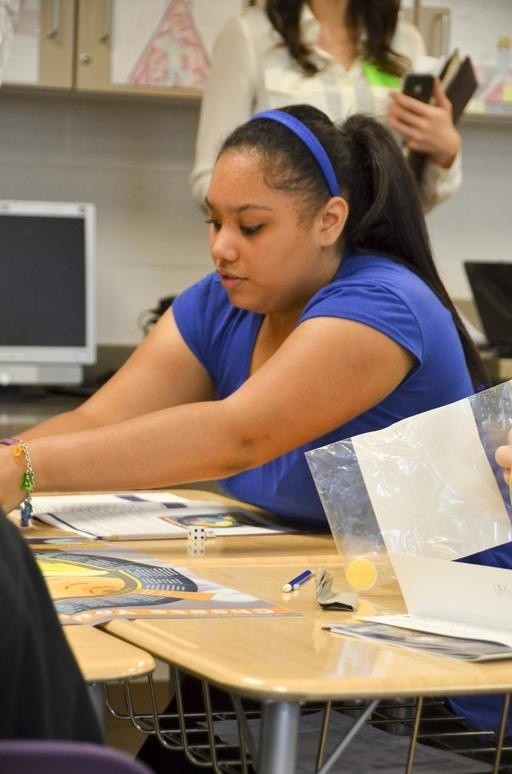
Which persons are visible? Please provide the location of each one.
[188,1,462,221]
[0,102,512,741]
[0,506,110,739]
[495,426,512,492]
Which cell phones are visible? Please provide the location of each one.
[398,73,435,126]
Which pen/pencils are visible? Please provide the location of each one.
[283,570,313,592]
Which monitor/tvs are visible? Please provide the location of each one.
[0,199,99,385]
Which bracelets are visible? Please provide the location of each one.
[0,438,35,528]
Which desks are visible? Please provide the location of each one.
[6,488,511,772]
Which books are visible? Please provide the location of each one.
[400,48,478,187]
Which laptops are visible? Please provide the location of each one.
[463,261,512,355]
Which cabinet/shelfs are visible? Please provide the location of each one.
[403,3,512,123]
[2,0,257,103]
